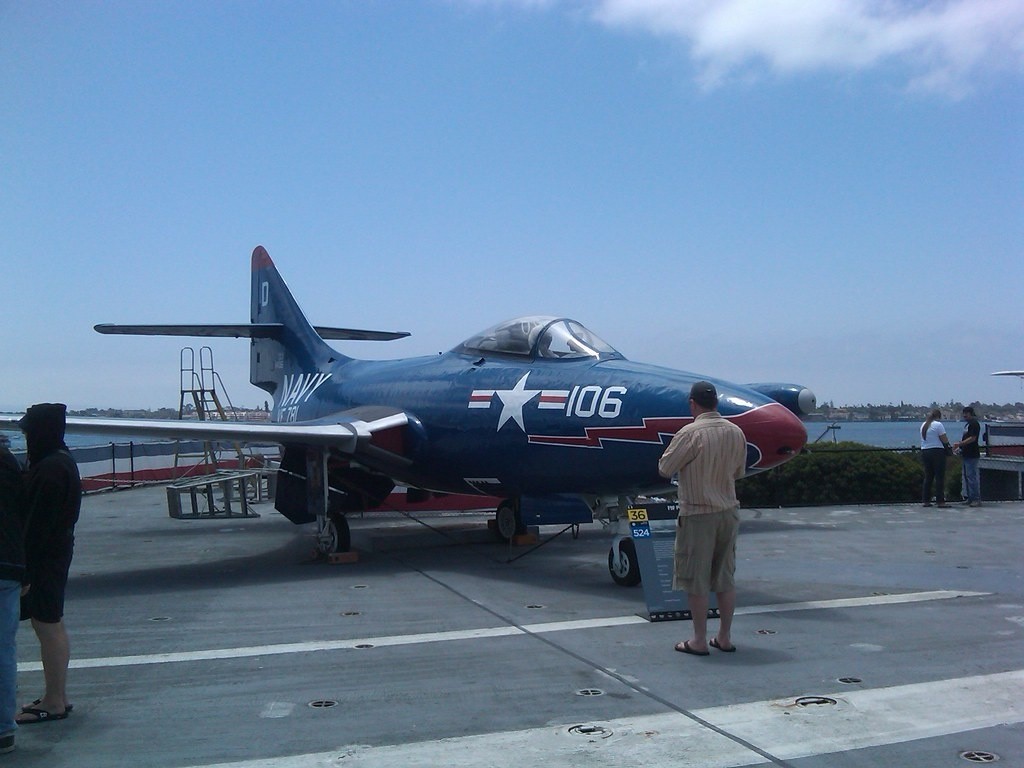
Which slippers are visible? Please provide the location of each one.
[15,709,68,723]
[675,640,709,655]
[710,638,736,652]
[22,698,72,710]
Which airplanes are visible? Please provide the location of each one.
[1,245,821,589]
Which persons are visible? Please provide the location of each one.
[920,407,981,508]
[659,380,747,656]
[527,325,559,358]
[0,404,81,754]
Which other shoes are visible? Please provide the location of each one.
[962,499,971,504]
[939,503,951,507]
[924,503,933,507]
[0,734,15,754]
[970,501,982,507]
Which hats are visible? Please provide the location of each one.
[11,413,27,433]
[690,381,717,399]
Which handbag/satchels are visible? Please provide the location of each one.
[945,442,953,456]
